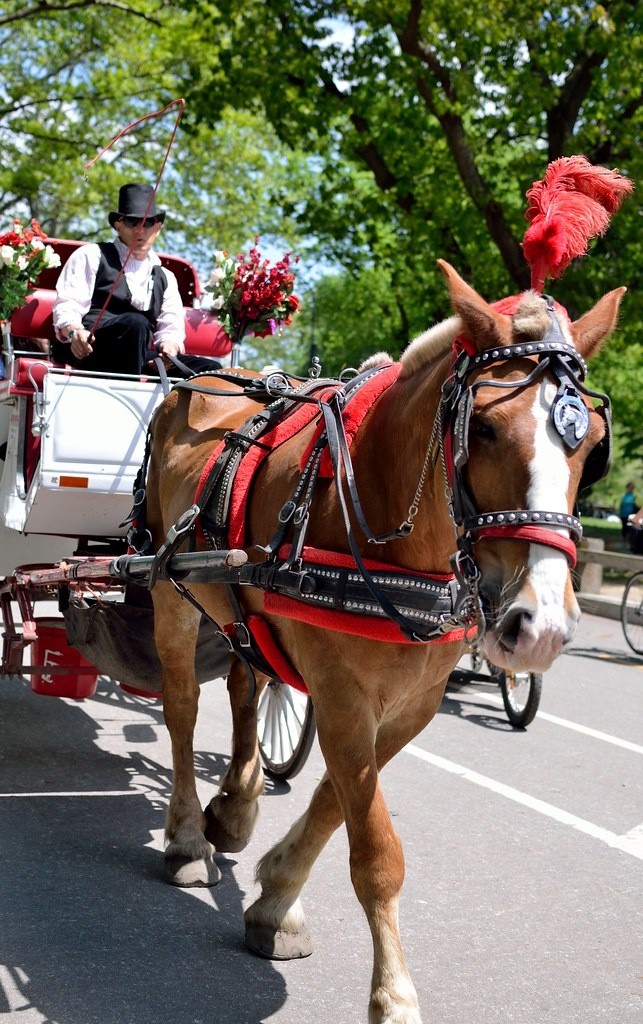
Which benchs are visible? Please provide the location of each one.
[5,357,157,490]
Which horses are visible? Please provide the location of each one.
[145,258,626,1023]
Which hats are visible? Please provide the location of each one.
[108,182,166,218]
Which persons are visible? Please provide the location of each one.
[619,482,640,544]
[52,183,224,383]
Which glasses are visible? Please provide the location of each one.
[119,216,162,228]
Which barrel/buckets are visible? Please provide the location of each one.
[31,619,96,696]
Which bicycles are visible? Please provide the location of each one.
[617,514,643,655]
[447,644,545,729]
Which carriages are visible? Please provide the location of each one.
[1,237,629,1024]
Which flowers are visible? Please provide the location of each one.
[203,232,300,341]
[0,217,60,323]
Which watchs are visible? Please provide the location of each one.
[67,329,77,343]
